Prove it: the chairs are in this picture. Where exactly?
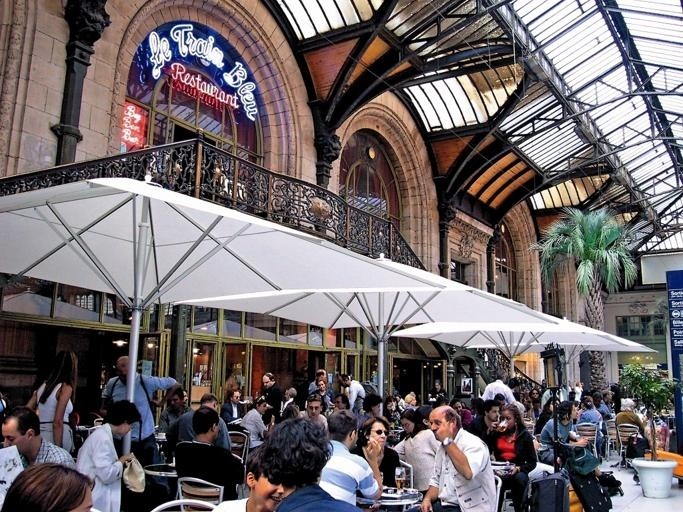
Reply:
[0,376,640,512]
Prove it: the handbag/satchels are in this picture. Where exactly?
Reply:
[363,380,378,395]
[597,471,621,496]
[626,436,650,459]
[123,453,146,493]
[575,447,600,475]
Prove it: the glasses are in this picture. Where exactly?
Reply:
[371,430,389,436]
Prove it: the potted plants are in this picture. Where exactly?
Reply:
[618,364,681,500]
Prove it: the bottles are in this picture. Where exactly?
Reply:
[203,378,210,387]
[194,373,198,385]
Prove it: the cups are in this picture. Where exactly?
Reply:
[394,466,406,495]
[372,472,383,484]
[496,419,508,432]
[394,418,398,429]
[157,433,164,438]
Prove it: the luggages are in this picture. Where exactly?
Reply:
[570,470,612,512]
[530,471,569,512]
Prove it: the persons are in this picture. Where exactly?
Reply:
[0,348,674,511]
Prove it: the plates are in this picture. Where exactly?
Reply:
[154,437,166,439]
[492,461,508,465]
